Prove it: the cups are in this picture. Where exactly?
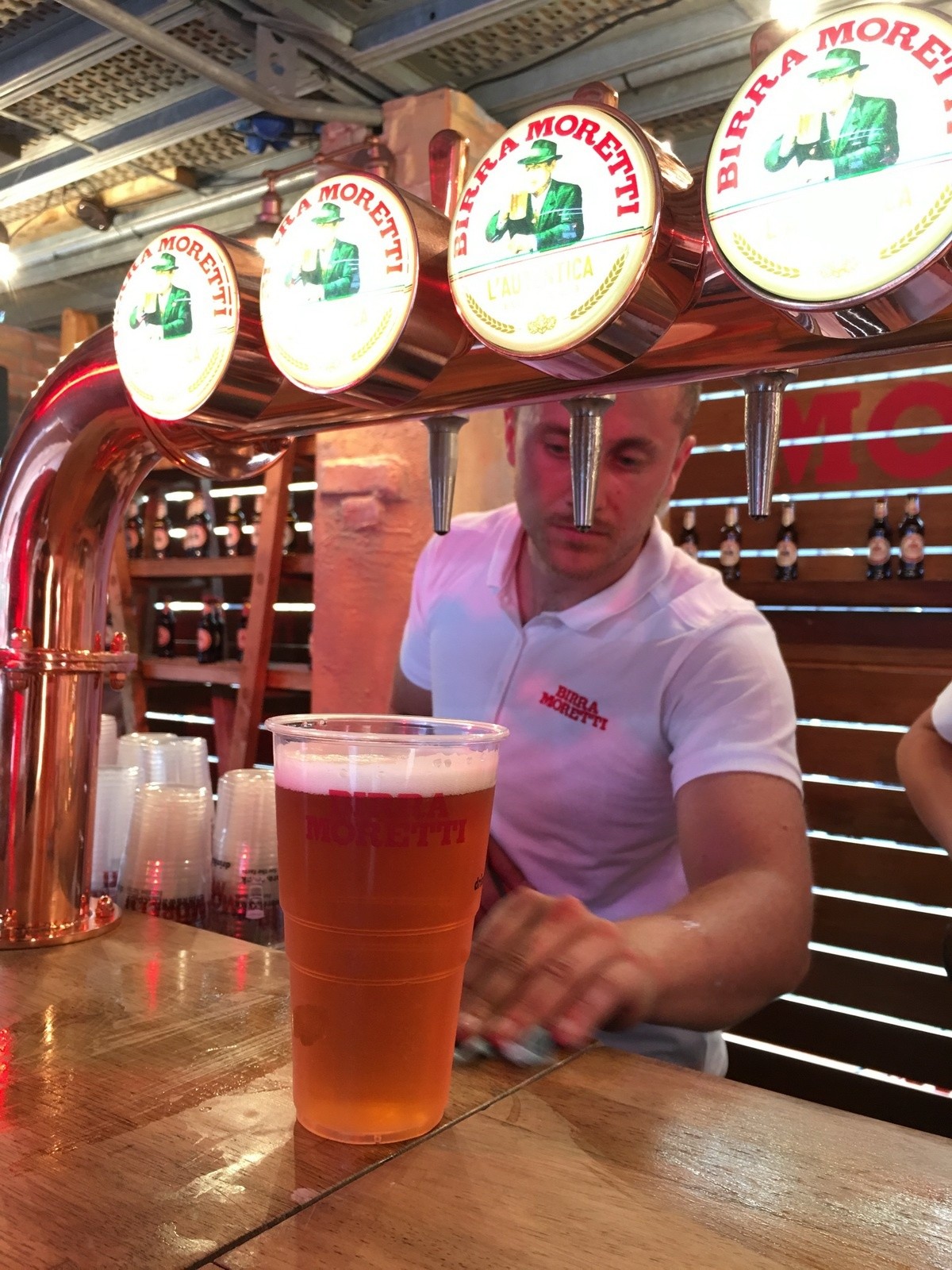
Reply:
[259,713,510,1145]
[90,714,284,951]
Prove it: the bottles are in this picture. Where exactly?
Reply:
[677,506,698,562]
[774,502,798,581]
[157,596,177,657]
[898,493,925,579]
[866,494,893,580]
[197,596,248,664]
[124,492,300,559]
[719,503,742,579]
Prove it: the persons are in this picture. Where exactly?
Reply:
[896,679,951,860]
[390,382,817,1079]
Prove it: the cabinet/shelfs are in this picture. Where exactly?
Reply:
[117,434,316,693]
